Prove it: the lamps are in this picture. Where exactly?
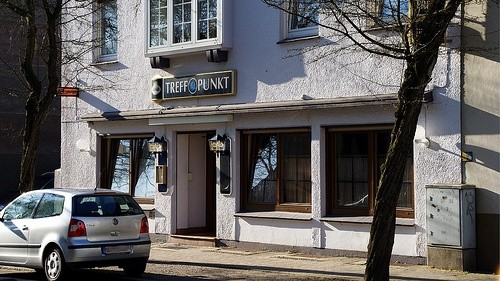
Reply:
[413,125,430,149]
[146,136,167,158]
[209,134,227,158]
[77,139,92,156]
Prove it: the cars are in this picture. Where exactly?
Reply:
[0,187,152,281]
